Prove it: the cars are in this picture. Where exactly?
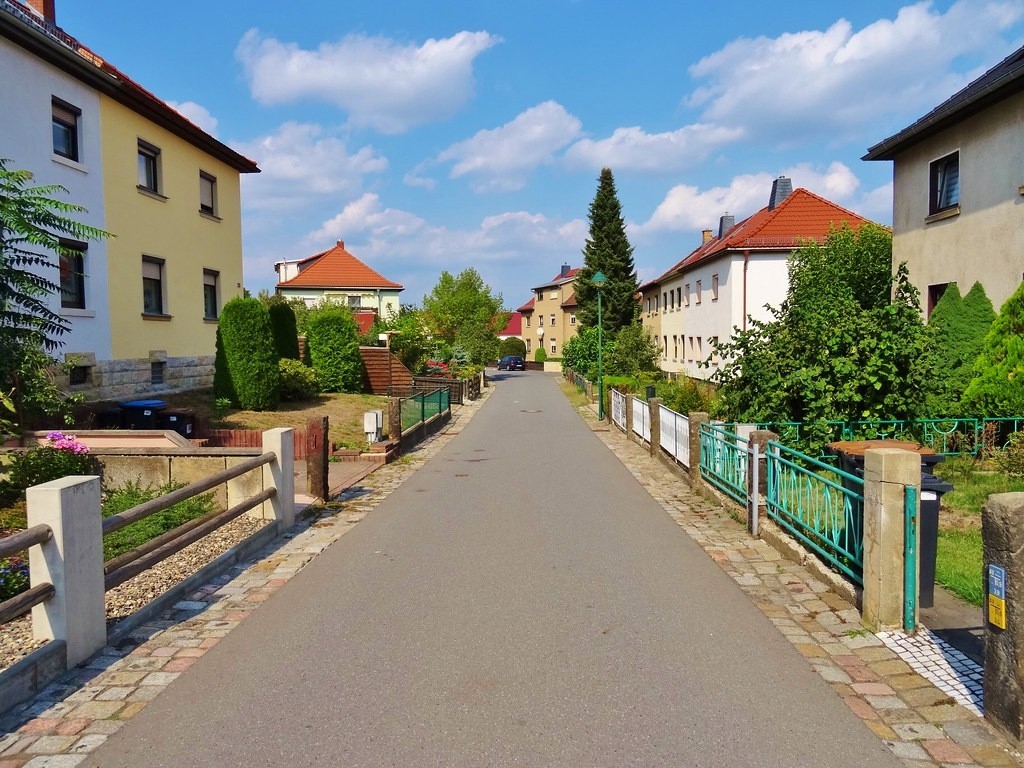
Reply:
[497,356,525,371]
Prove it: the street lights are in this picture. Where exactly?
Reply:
[592,272,607,420]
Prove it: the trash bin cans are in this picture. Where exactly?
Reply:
[855,467,955,609]
[95,408,125,430]
[158,407,195,439]
[824,438,946,592]
[119,399,167,430]
